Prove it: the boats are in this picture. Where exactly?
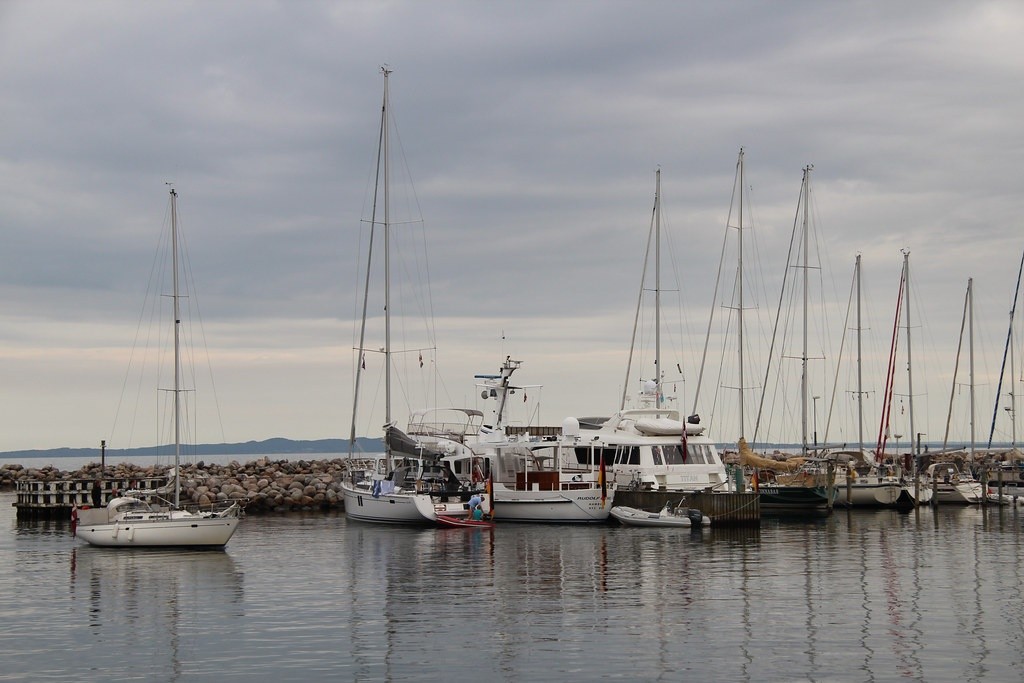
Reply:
[610,496,711,527]
[339,63,1024,525]
[72,182,241,547]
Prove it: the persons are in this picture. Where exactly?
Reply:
[487,472,495,519]
[473,506,483,520]
[92,479,101,508]
[442,467,460,502]
[467,497,485,518]
[125,480,136,491]
[108,489,117,503]
[473,461,484,481]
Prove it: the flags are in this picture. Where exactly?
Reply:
[683,422,687,463]
[598,455,607,507]
[753,470,760,493]
